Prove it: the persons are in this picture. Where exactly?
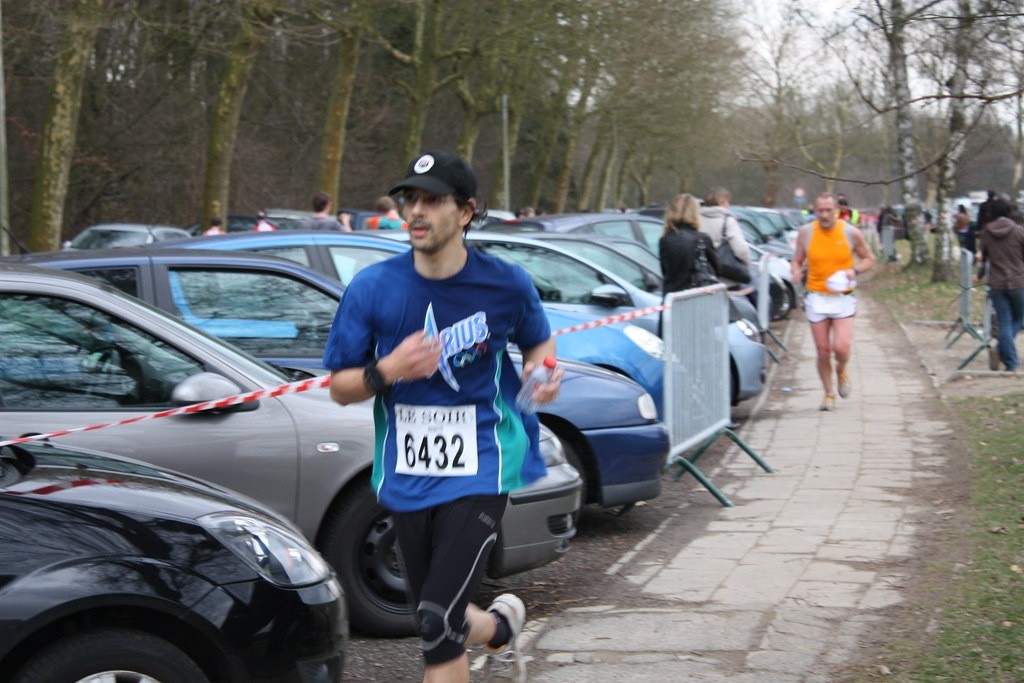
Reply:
[203,219,225,236]
[656,193,741,428]
[301,193,352,233]
[367,196,408,229]
[515,203,547,219]
[323,151,563,683]
[253,212,276,233]
[955,188,1024,372]
[791,193,876,411]
[697,186,751,295]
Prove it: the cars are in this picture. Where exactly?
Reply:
[132,229,691,432]
[482,206,818,408]
[0,252,667,531]
[63,224,190,259]
[339,207,515,230]
[204,211,344,232]
[0,260,588,631]
[0,438,349,683]
[857,192,988,226]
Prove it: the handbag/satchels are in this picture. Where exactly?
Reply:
[690,239,718,290]
[713,215,752,284]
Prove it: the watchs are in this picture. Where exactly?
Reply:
[363,360,397,395]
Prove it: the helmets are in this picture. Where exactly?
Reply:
[389,150,478,199]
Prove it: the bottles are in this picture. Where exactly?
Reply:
[516,356,556,412]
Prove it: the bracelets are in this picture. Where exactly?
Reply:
[852,267,860,276]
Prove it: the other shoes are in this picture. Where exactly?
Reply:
[989,347,1001,370]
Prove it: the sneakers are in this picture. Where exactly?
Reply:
[484,594,527,683]
[838,370,851,398]
[820,394,836,412]
